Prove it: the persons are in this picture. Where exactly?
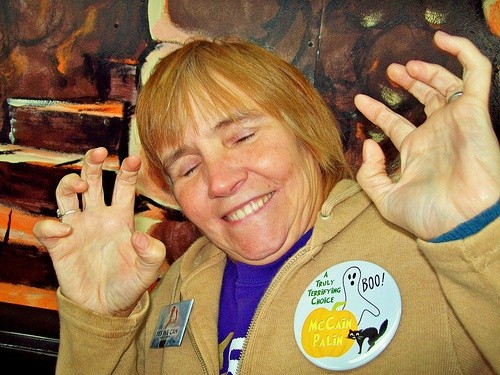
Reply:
[447,92,463,104]
[33,30,499,375]
[54,206,79,218]
